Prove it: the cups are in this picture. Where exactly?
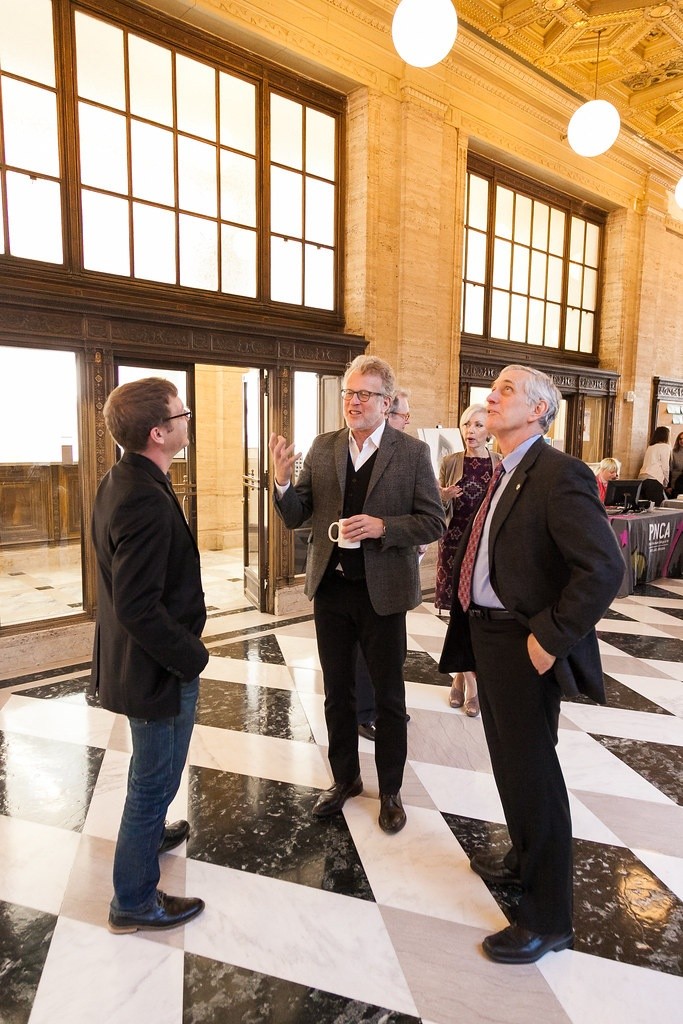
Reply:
[328,519,360,548]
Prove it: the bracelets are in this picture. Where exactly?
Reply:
[381,527,386,538]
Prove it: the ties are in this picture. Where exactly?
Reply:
[457,461,506,613]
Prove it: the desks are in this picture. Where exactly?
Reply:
[608,499,683,600]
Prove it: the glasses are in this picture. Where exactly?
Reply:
[390,410,411,421]
[340,389,386,402]
[161,406,192,423]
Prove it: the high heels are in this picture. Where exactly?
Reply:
[464,691,480,717]
[449,676,465,708]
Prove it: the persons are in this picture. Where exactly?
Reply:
[596,458,620,509]
[436,365,625,965]
[91,379,209,934]
[666,432,683,496]
[356,391,411,744]
[437,403,503,717]
[269,357,447,836]
[638,427,673,508]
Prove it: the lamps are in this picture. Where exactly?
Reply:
[392,0,458,67]
[674,174,683,209]
[567,27,620,157]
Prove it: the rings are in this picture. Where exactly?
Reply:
[361,528,364,533]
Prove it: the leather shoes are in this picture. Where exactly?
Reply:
[312,773,364,817]
[482,919,574,964]
[108,889,205,935]
[155,819,190,854]
[358,724,376,741]
[406,713,410,722]
[469,852,526,885]
[378,789,407,834]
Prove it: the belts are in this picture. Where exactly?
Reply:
[465,608,516,621]
[334,569,366,580]
